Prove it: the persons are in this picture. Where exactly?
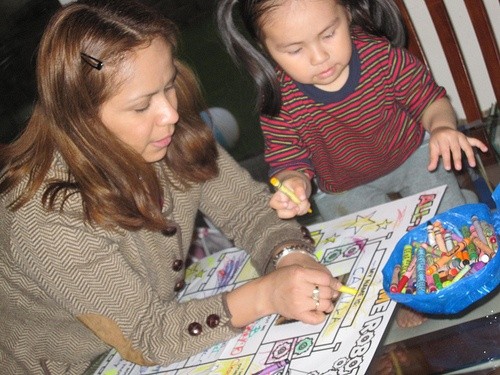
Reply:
[218,0,487,219]
[0,0,341,375]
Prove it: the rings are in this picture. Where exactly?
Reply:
[312,285,320,310]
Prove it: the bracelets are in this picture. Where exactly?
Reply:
[272,244,319,271]
